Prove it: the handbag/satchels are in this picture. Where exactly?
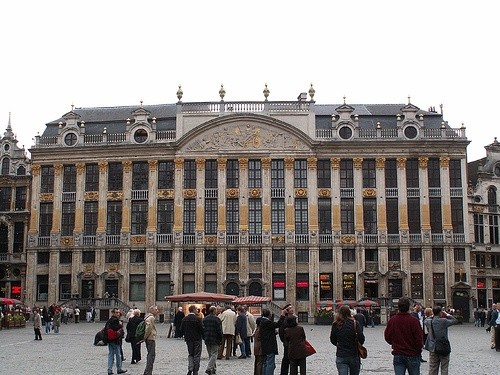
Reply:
[359,346,367,359]
[94,331,107,346]
[435,339,451,357]
[305,339,316,357]
[236,333,242,344]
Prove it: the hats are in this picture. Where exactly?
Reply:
[282,304,292,311]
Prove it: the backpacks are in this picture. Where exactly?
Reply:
[135,315,151,340]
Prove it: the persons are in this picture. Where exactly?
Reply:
[253,303,306,375]
[215,303,256,360]
[105,304,144,375]
[0,304,80,334]
[411,304,434,363]
[384,298,423,375]
[474,308,480,327]
[33,310,42,340]
[486,303,500,350]
[349,305,375,332]
[181,305,203,375]
[141,306,159,375]
[424,306,456,375]
[330,306,365,375]
[202,306,222,375]
[174,307,185,339]
[480,309,486,327]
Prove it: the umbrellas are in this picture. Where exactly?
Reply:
[317,299,379,307]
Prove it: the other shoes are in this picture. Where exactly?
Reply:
[205,370,212,375]
[217,354,251,360]
[121,357,126,361]
[117,369,127,374]
[212,371,216,374]
[474,324,484,327]
[420,356,427,362]
[131,360,136,364]
[187,370,192,375]
[108,372,114,375]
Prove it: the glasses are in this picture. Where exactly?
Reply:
[115,312,120,314]
